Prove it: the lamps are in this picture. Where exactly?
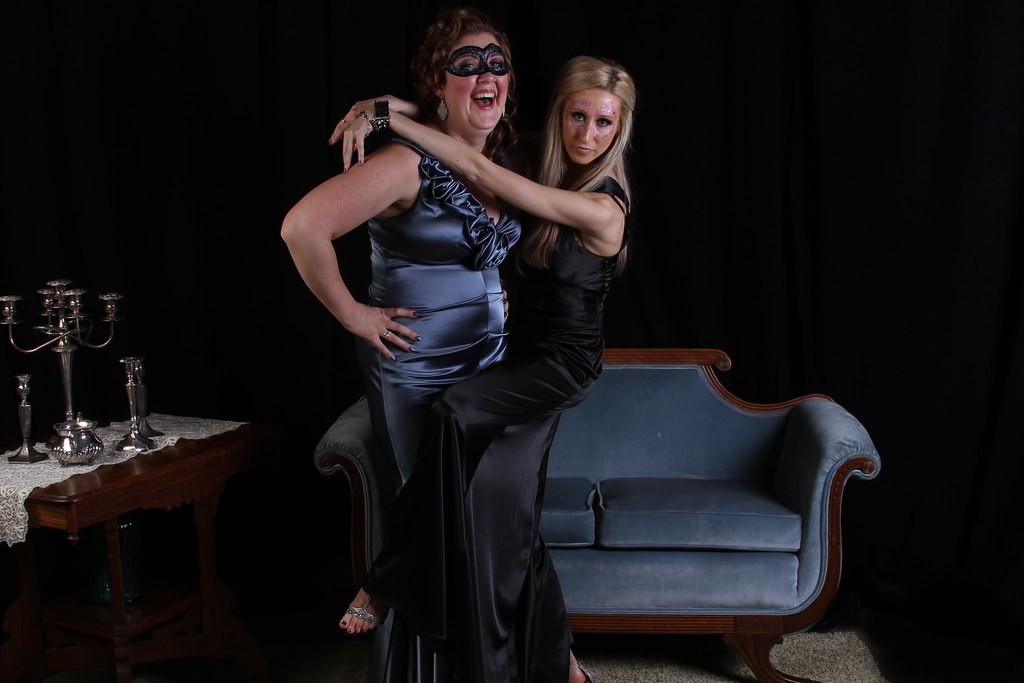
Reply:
[1,282,120,423]
[117,360,154,454]
[10,374,46,464]
[131,358,163,438]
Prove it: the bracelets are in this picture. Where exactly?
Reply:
[359,99,391,137]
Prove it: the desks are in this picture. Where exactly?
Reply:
[0,413,276,683]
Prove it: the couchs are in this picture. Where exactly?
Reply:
[314,351,880,682]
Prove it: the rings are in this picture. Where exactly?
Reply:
[342,118,346,124]
[383,330,391,341]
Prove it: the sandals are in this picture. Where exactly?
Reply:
[339,593,387,637]
[568,660,596,683]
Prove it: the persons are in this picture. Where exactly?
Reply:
[280,9,526,683]
[335,55,638,683]
